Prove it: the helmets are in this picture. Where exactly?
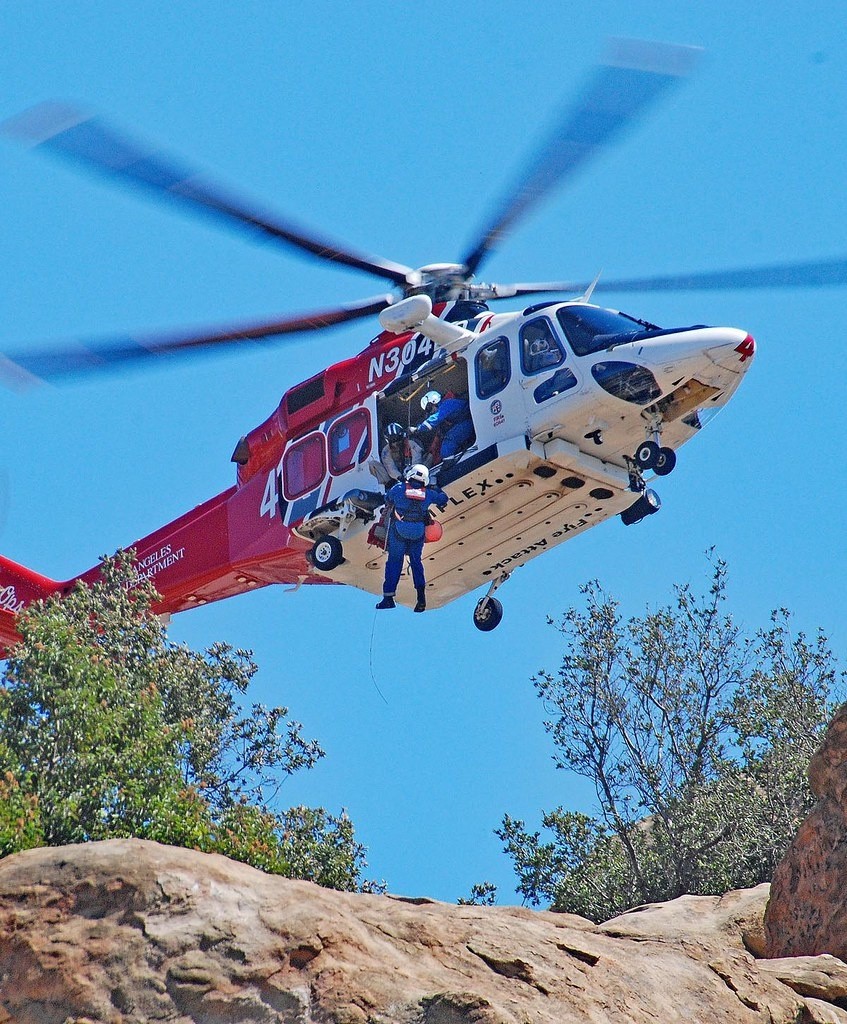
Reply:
[420,390,441,415]
[406,464,430,487]
[384,423,404,442]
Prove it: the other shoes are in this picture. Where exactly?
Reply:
[375,595,396,609]
[413,601,426,613]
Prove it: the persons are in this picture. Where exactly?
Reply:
[369,423,434,491]
[562,312,618,352]
[406,391,474,472]
[529,330,576,399]
[375,464,448,613]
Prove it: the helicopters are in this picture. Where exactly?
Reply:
[2,26,846,678]
